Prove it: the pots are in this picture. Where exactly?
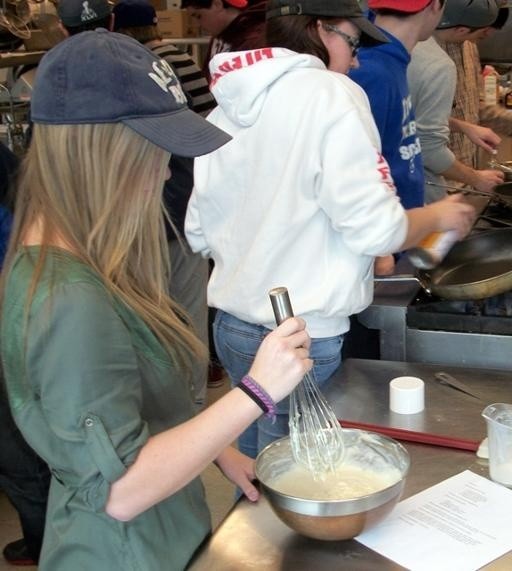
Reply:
[369,229,512,300]
[426,178,512,207]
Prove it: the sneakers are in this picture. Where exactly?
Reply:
[2,539,35,565]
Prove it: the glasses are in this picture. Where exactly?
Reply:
[320,24,360,57]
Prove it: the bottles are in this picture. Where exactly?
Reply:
[487,149,500,170]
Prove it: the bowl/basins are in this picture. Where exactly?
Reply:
[252,427,411,546]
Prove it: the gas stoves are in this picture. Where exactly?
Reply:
[401,182,508,334]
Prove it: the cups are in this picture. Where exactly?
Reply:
[482,401,512,484]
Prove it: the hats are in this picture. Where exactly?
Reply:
[368,0,431,14]
[31,29,232,157]
[58,1,110,27]
[112,0,158,27]
[264,1,393,51]
[437,2,498,31]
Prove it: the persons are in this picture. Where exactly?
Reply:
[2,4,512,407]
[2,23,318,571]
[2,141,54,568]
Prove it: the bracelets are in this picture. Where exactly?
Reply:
[241,378,274,411]
[243,375,277,408]
[236,382,269,415]
